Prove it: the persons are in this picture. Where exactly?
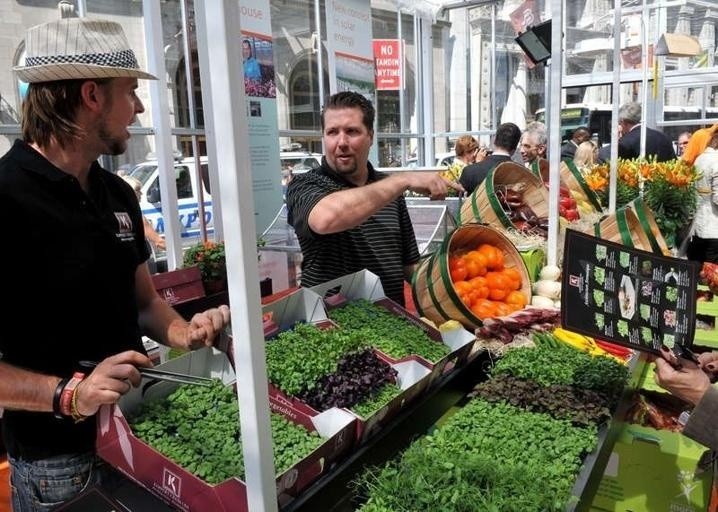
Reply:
[0,21,230,511]
[520,121,546,167]
[243,40,261,83]
[653,345,718,457]
[561,103,692,164]
[690,123,718,276]
[456,124,520,197]
[286,91,465,311]
[124,177,167,251]
[446,133,488,186]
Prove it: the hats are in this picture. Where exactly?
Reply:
[12,17,160,83]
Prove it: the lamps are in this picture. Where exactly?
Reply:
[657,32,702,56]
[514,19,565,66]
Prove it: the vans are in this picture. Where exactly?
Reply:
[121,151,320,255]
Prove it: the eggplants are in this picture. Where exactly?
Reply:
[474,304,562,344]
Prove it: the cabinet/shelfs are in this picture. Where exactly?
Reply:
[50,219,716,512]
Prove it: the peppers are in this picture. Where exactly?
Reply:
[557,185,582,224]
[446,242,531,320]
[497,183,550,241]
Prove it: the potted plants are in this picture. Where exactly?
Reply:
[183,236,265,293]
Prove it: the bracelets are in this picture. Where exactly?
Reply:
[52,362,96,424]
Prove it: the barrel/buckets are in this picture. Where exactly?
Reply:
[410,222,532,335]
[456,160,550,239]
[525,159,550,186]
[583,196,670,255]
[559,160,603,216]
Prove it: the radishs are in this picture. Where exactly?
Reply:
[530,262,561,311]
